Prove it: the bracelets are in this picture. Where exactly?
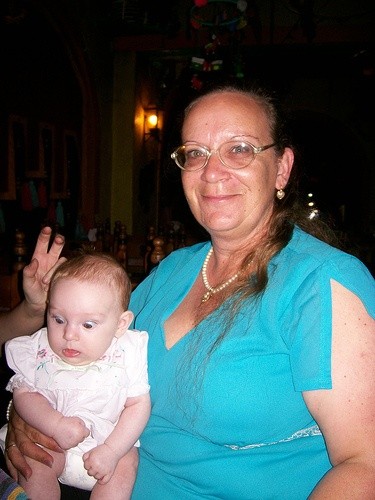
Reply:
[3,396,17,428]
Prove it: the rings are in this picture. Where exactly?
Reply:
[3,442,17,452]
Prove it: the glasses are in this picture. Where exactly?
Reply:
[170,142,278,173]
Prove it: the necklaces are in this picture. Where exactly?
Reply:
[200,246,263,303]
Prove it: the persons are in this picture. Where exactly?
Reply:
[3,223,71,346]
[1,79,374,499]
[5,250,154,498]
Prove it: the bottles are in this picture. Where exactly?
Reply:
[9,232,30,310]
[73,220,191,279]
